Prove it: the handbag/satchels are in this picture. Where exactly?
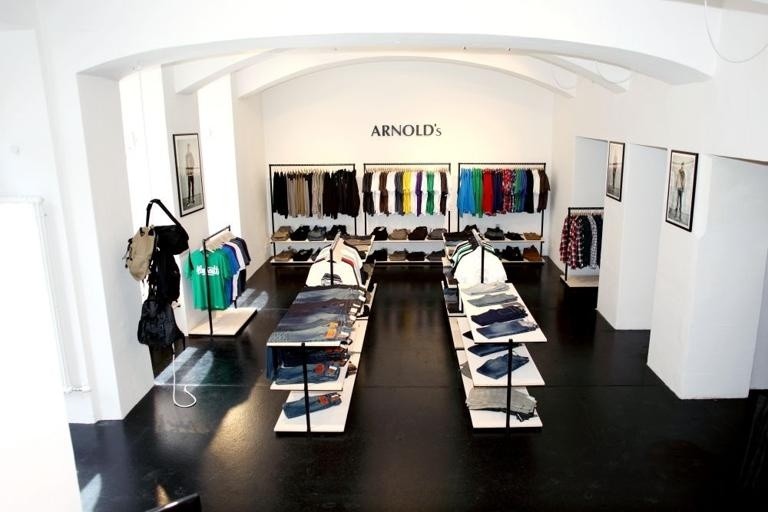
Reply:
[144,198,191,255]
[134,298,185,352]
[124,225,157,283]
[148,252,181,305]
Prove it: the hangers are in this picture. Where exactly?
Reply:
[199,228,237,253]
[570,207,604,218]
[460,162,546,176]
[364,162,451,174]
[273,163,355,176]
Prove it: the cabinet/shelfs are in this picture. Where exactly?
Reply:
[440,231,548,440]
[272,232,381,441]
[381,232,440,266]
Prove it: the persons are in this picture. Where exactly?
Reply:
[609,147,619,193]
[673,161,686,222]
[183,143,197,208]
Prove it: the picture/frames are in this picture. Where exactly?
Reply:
[605,140,625,202]
[173,132,205,217]
[665,149,700,232]
